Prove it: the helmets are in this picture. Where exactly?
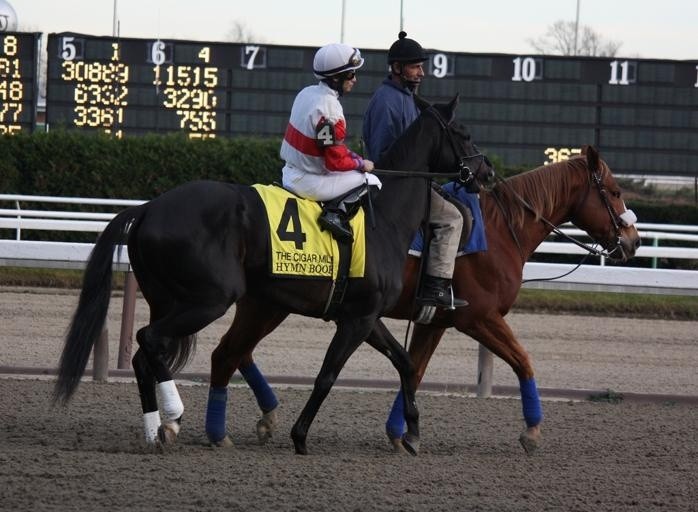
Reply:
[388,31,429,64]
[313,43,365,80]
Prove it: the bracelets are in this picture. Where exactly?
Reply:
[356,157,365,171]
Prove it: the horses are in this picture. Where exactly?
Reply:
[205,142,646,460]
[54,91,496,458]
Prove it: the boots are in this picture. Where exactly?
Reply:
[318,183,371,242]
[419,275,469,306]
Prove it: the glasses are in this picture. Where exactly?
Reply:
[320,71,355,80]
[313,48,361,73]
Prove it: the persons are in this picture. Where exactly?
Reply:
[279,41,383,244]
[361,31,470,309]
